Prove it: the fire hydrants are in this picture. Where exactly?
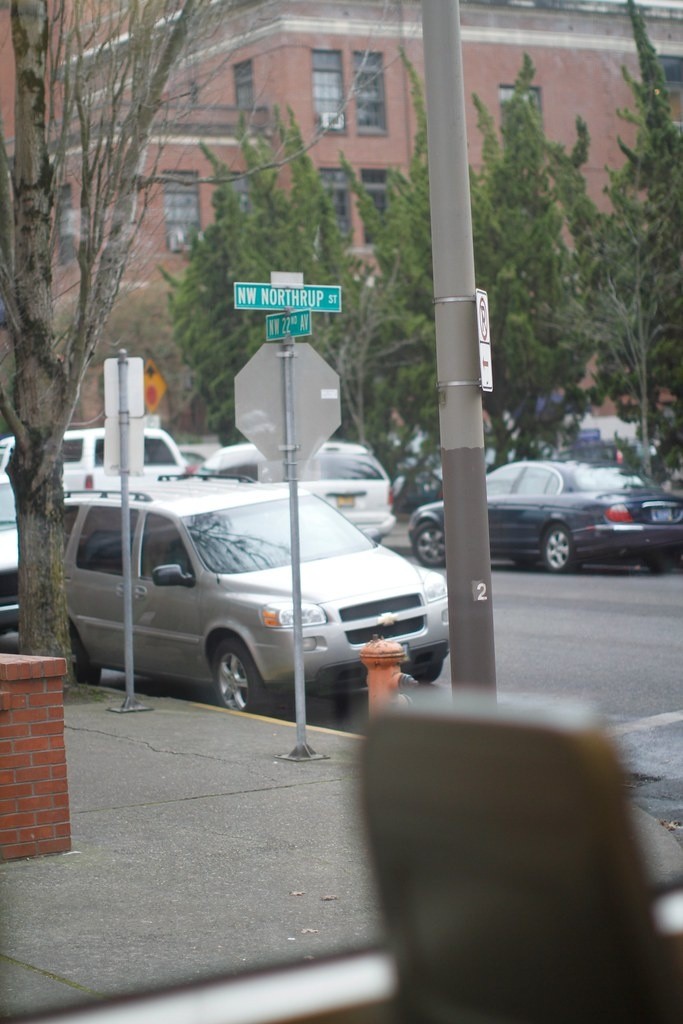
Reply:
[359,634,420,721]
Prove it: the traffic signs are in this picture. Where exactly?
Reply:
[264,308,312,342]
[233,280,342,313]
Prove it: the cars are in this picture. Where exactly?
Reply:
[408,454,683,571]
[188,437,397,545]
[0,474,20,634]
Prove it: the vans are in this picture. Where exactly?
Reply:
[0,425,194,488]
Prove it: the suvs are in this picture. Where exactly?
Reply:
[60,474,450,713]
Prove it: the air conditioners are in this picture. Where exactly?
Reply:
[172,232,204,252]
[321,112,345,129]
